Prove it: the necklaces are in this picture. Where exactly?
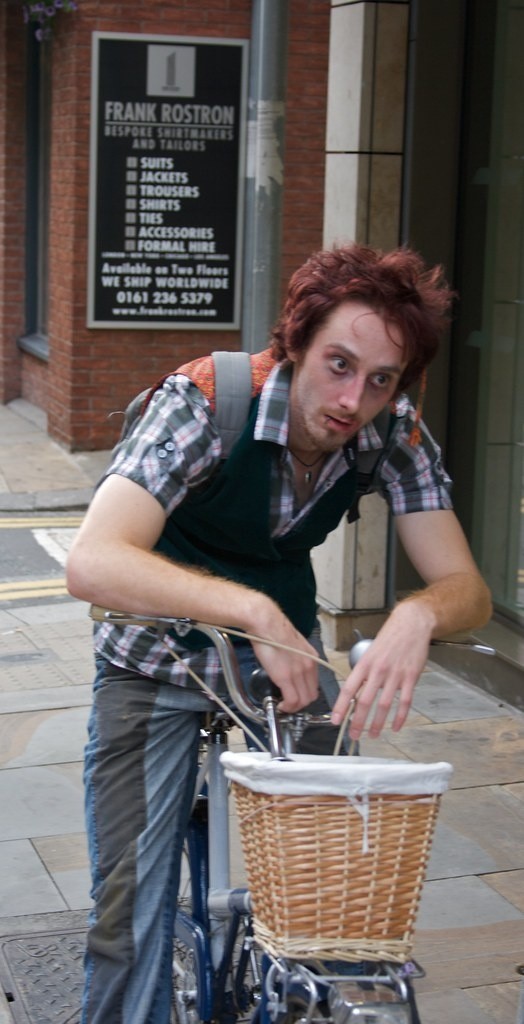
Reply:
[288,448,324,484]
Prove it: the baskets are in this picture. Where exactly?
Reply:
[219,742,452,964]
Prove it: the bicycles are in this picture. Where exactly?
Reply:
[84,599,456,1024]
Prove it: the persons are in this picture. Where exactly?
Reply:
[66,243,492,1024]
